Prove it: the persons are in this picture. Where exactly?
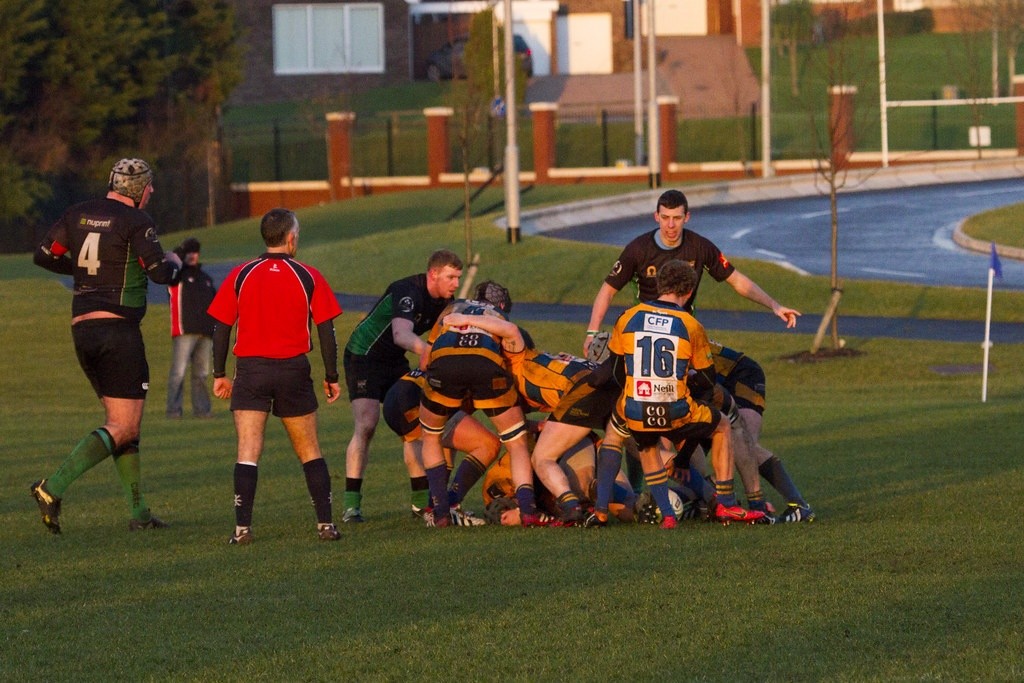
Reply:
[167,238,217,420]
[207,209,342,544]
[343,189,815,527]
[30,157,187,535]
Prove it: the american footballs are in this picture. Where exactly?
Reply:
[655,488,684,523]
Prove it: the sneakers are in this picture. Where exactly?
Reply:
[228,526,253,545]
[549,505,583,527]
[714,503,766,527]
[570,511,607,528]
[30,477,64,537]
[519,512,561,529]
[749,511,781,524]
[411,503,432,522]
[425,515,451,528]
[702,492,718,523]
[450,504,485,526]
[316,522,339,540]
[129,508,169,532]
[778,502,815,524]
[657,516,679,530]
[342,507,365,523]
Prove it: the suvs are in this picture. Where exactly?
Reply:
[425,31,534,84]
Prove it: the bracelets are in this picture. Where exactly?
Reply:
[584,329,599,338]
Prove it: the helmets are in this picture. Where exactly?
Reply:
[475,280,512,314]
[587,332,613,365]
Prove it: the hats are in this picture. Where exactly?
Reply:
[107,158,154,200]
[181,239,200,253]
